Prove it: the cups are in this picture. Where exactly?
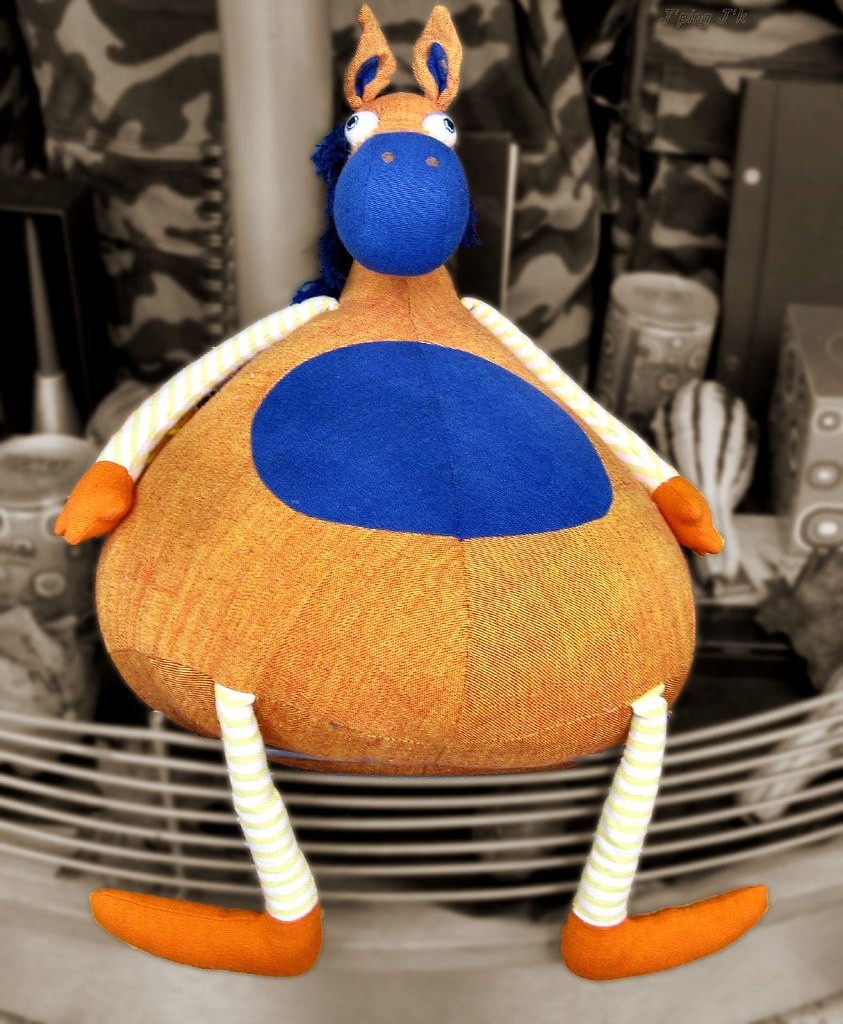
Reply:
[0,433,108,633]
[598,269,720,429]
